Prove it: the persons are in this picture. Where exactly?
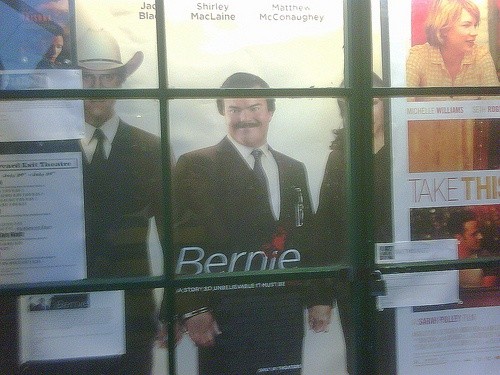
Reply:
[169,72,334,375]
[75,29,185,375]
[439,210,497,290]
[316,69,390,375]
[406,0,499,88]
[25,21,70,66]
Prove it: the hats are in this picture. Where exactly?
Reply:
[78,26,143,78]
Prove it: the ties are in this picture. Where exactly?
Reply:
[251,149,269,208]
[89,129,108,170]
